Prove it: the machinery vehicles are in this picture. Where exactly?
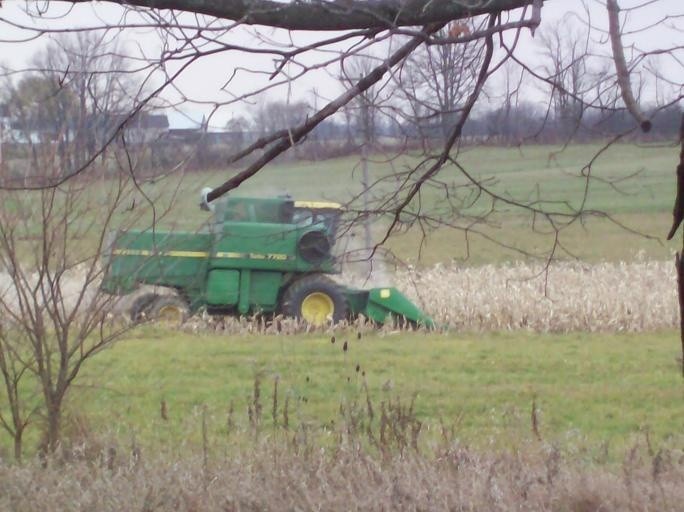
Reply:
[97,191,435,337]
